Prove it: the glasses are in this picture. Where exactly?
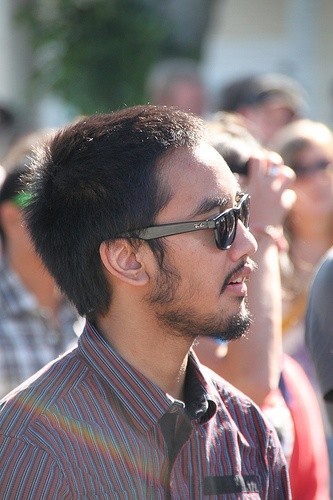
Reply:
[114,190,250,250]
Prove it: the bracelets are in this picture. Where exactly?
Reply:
[250,222,289,252]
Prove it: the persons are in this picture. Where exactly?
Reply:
[0,106,292,500]
[0,130,87,401]
[266,119,333,500]
[190,128,329,500]
[223,71,307,146]
